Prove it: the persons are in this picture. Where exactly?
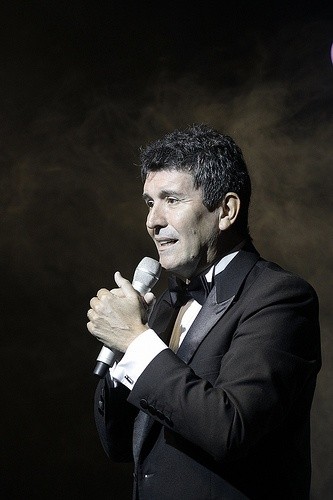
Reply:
[88,126,322,500]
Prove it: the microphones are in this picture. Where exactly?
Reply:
[92,257,162,378]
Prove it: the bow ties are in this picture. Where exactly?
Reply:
[167,271,210,307]
